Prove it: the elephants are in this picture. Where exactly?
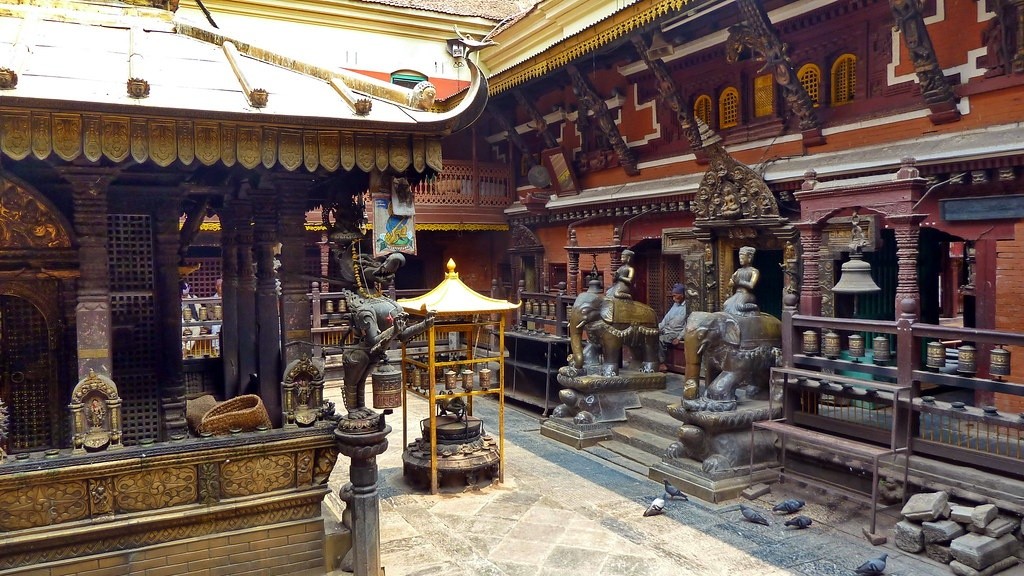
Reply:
[566,292,659,376]
[683,312,783,399]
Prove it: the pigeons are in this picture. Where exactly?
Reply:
[784,516,812,528]
[855,554,889,573]
[739,504,769,526]
[662,478,688,501]
[643,492,666,516]
[772,499,805,513]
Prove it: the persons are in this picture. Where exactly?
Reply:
[413,81,436,109]
[90,399,102,427]
[298,381,309,405]
[182,282,207,359]
[605,249,635,300]
[249,88,269,106]
[354,98,372,113]
[126,77,150,97]
[720,187,737,211]
[658,283,687,373]
[502,0,929,155]
[723,246,761,317]
[211,278,222,356]
[0,66,18,88]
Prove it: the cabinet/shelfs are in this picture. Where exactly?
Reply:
[751,368,913,535]
[494,332,571,418]
